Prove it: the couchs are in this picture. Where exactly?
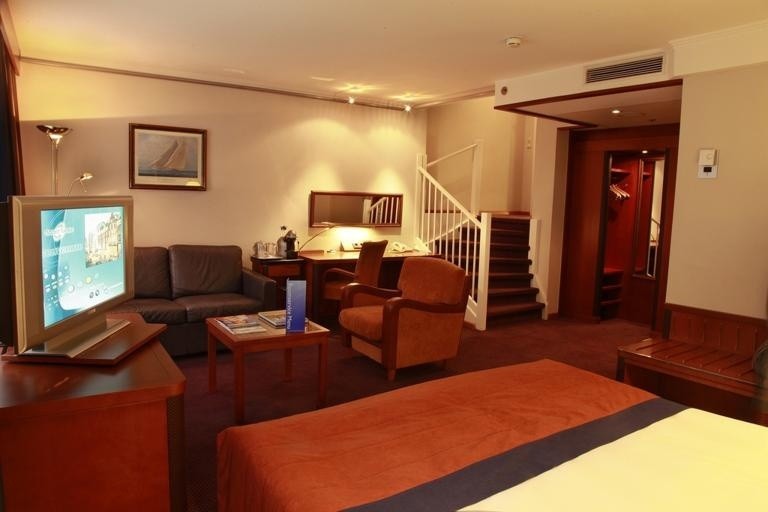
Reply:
[129,243,278,358]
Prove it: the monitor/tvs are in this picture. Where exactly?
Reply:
[0,195,135,359]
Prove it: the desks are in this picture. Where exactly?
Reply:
[251,255,304,279]
[297,249,442,322]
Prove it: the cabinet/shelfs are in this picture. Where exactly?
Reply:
[600,268,624,305]
[0,312,190,511]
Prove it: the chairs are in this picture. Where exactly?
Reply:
[321,239,472,388]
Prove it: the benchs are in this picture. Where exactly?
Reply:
[616,303,767,403]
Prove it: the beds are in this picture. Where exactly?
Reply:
[214,357,767,512]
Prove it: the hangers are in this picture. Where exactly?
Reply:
[609,183,631,201]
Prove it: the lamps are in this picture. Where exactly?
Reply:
[37,124,94,195]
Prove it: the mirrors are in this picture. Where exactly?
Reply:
[310,191,403,228]
[630,155,666,280]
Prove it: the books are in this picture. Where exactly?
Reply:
[258,308,309,329]
[214,314,266,335]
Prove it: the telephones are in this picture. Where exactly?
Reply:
[392,242,413,253]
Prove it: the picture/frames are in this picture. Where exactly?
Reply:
[128,122,208,192]
